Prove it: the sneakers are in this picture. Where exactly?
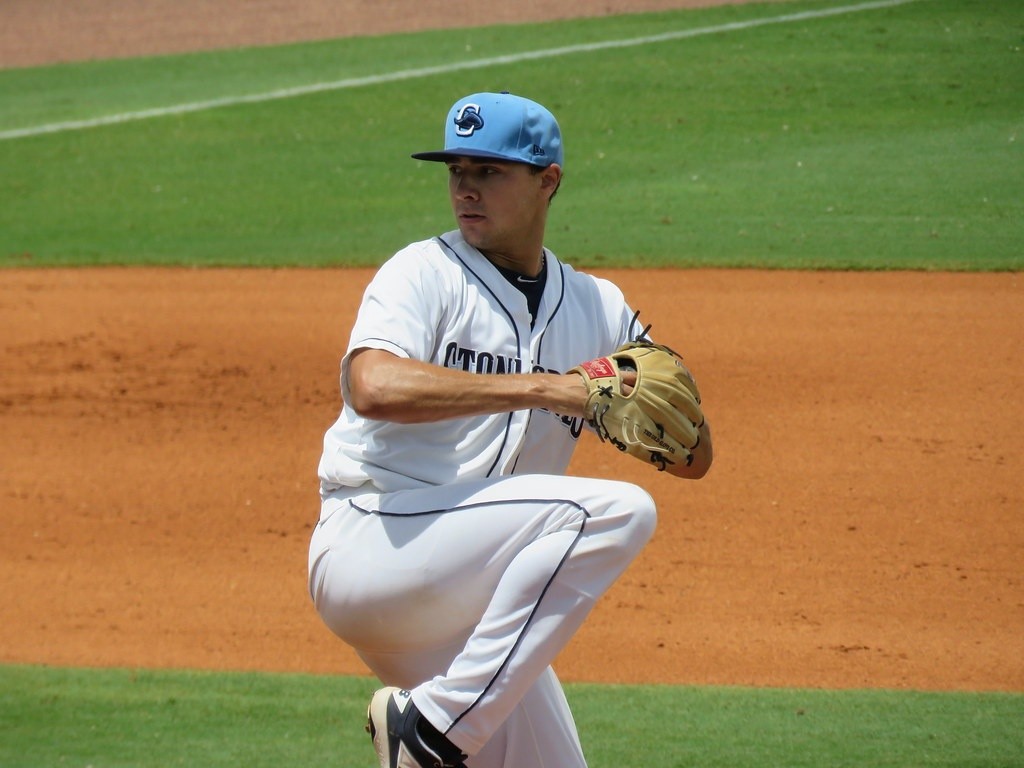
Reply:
[365,688,468,768]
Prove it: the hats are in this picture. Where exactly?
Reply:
[410,92,563,170]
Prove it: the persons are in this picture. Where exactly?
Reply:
[305,92,713,768]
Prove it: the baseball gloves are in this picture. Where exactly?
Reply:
[564,342,703,471]
